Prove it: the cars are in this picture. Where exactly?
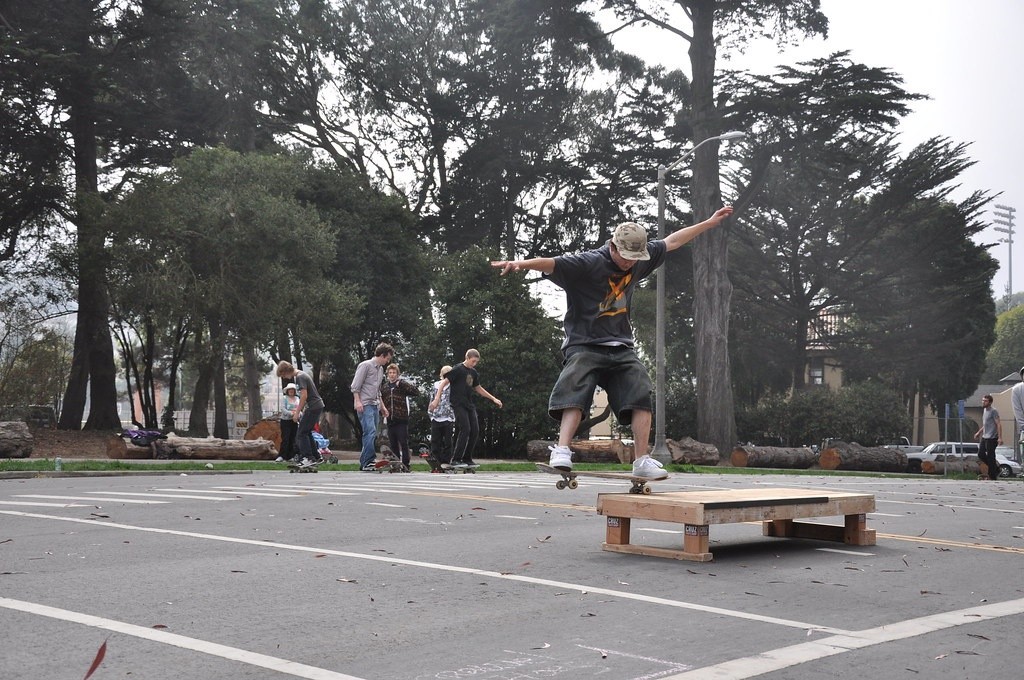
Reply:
[806,433,1023,478]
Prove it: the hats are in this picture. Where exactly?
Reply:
[612,222,651,261]
[283,383,297,394]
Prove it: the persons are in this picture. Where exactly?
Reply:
[276,383,300,462]
[277,360,325,468]
[974,395,1004,480]
[491,206,733,480]
[381,363,420,473]
[351,343,394,471]
[428,366,455,463]
[431,349,502,466]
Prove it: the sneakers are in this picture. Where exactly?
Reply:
[451,460,468,467]
[548,443,575,471]
[633,457,668,479]
[464,460,479,468]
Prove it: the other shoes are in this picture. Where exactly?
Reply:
[361,459,379,471]
[288,457,294,461]
[315,457,325,464]
[276,456,283,462]
[296,457,317,467]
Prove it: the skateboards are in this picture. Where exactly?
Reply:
[380,444,408,473]
[417,443,446,473]
[440,463,481,474]
[363,460,390,473]
[287,465,318,473]
[533,462,671,495]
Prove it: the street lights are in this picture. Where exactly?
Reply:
[650,130,750,464]
[994,202,1018,312]
